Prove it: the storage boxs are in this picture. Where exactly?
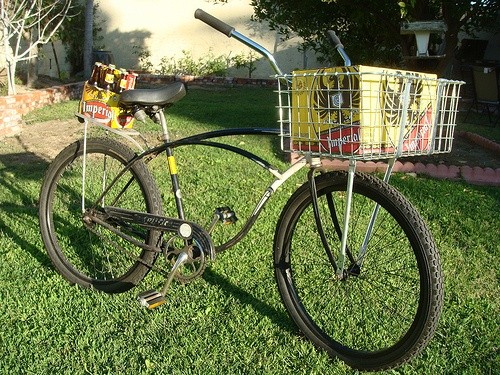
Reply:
[289,65,438,156]
[78,80,136,130]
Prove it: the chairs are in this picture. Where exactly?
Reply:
[463,66,500,127]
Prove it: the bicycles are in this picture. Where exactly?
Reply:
[38,8,468,371]
